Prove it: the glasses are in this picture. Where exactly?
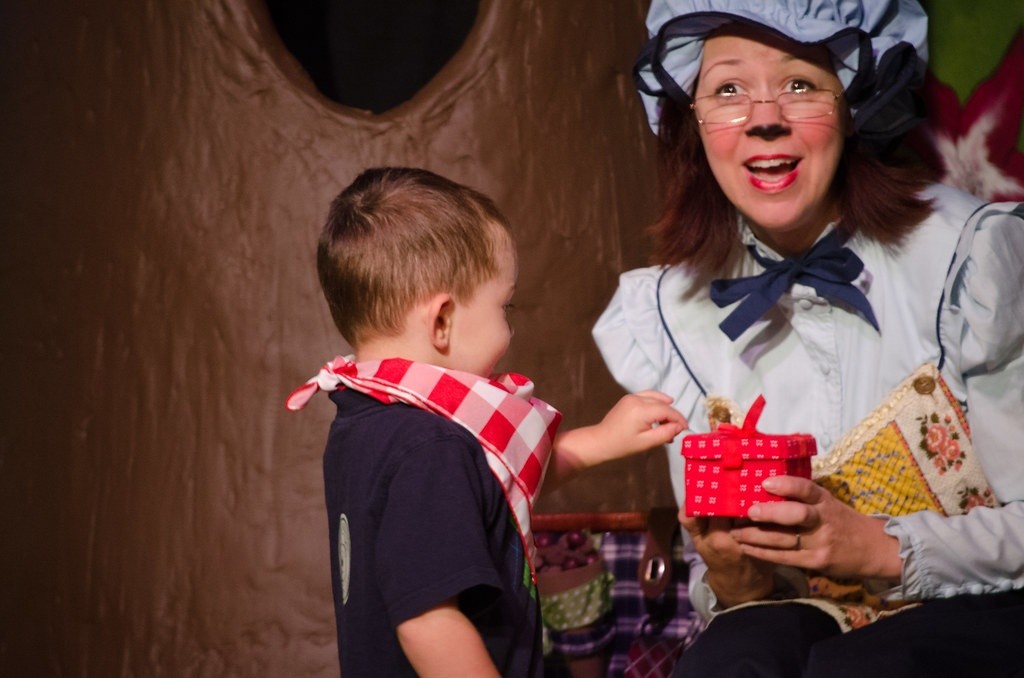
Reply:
[688,88,850,126]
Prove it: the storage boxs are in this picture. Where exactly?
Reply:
[680,431,817,519]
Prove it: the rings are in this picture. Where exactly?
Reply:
[795,533,801,549]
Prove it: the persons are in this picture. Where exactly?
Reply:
[591,0,1024,678]
[315,166,689,678]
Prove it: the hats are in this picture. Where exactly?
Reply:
[632,1,931,137]
